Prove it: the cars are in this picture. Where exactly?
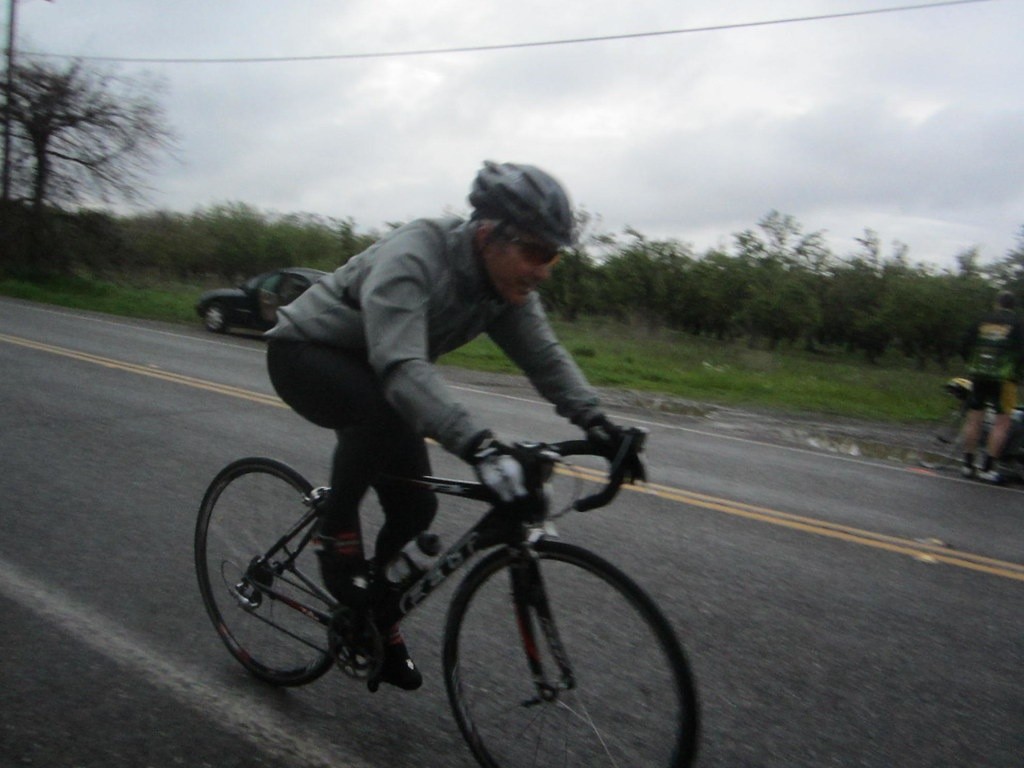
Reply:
[194,268,336,334]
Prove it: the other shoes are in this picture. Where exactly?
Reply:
[960,464,999,481]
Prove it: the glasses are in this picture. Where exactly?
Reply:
[511,234,559,266]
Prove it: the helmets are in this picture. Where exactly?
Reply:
[471,163,574,253]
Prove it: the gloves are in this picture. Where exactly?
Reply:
[593,419,644,476]
[472,442,550,524]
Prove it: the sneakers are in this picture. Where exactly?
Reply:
[314,509,366,601]
[374,625,425,693]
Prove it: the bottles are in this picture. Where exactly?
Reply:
[385,532,443,584]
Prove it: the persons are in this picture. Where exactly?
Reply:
[262,160,649,693]
[960,292,1024,483]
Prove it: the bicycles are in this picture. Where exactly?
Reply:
[923,374,1023,486]
[192,423,701,768]
